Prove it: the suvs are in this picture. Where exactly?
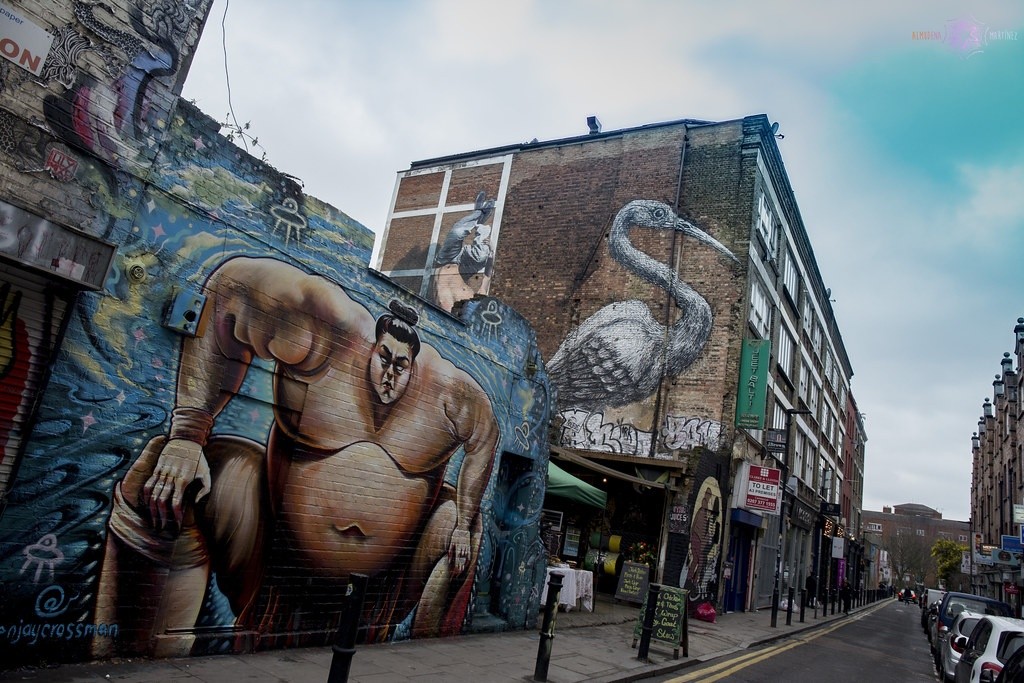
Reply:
[930,591,1013,666]
[921,589,958,629]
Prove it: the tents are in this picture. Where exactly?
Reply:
[546,460,607,612]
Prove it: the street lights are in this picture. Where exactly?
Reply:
[770,410,813,627]
[961,521,973,593]
[857,529,872,606]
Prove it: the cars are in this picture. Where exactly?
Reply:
[925,600,966,642]
[939,610,987,683]
[898,589,916,603]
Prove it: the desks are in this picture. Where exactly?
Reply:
[539,567,593,614]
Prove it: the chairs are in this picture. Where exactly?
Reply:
[948,604,964,619]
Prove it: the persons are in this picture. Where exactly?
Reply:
[903,586,911,607]
[806,571,817,609]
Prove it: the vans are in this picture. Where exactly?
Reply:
[953,615,1024,683]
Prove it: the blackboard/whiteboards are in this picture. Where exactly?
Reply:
[635,582,688,649]
[615,560,652,603]
